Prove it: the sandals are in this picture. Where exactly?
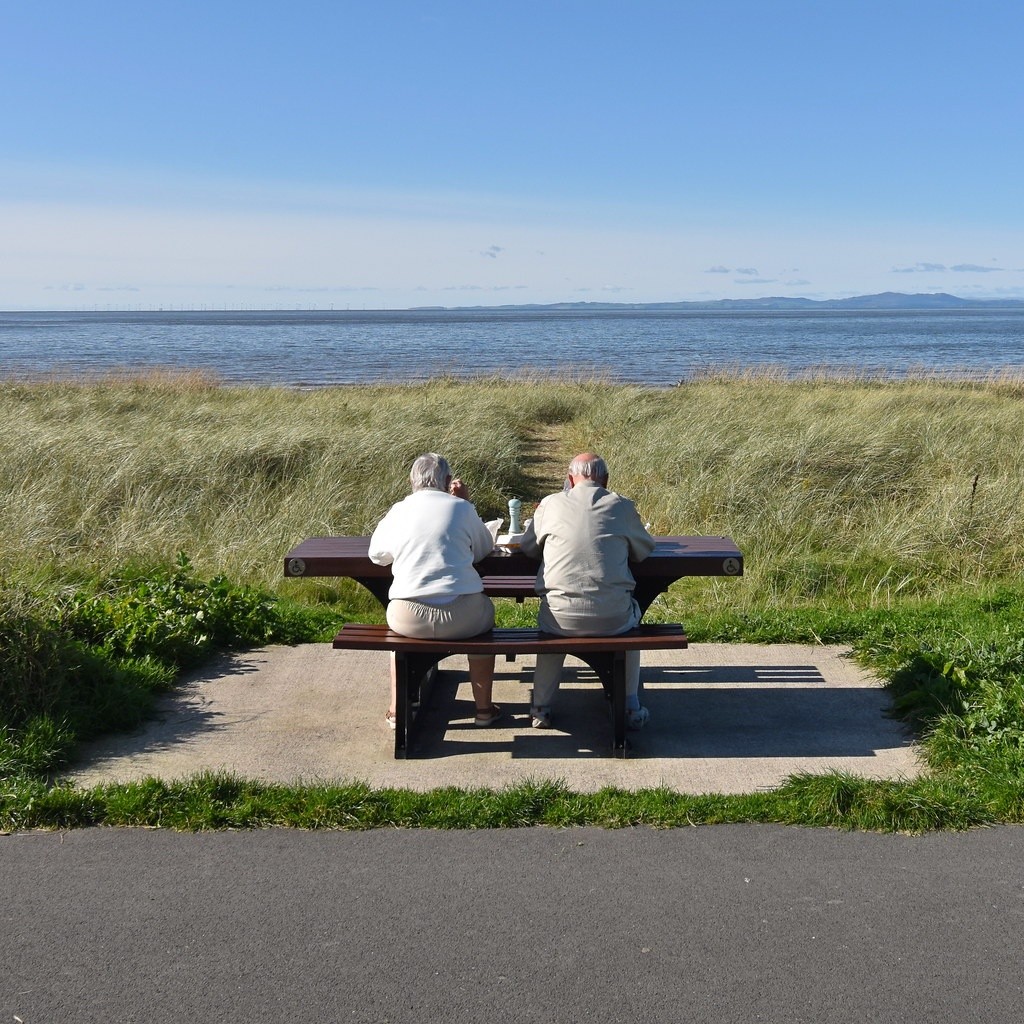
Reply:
[474,703,504,726]
[384,710,397,731]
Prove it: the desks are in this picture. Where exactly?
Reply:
[283,531,744,713]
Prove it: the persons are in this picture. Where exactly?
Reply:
[367,449,504,729]
[521,452,656,731]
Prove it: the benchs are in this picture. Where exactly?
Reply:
[478,575,542,604]
[331,620,689,761]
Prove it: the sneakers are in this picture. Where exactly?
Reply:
[627,706,651,730]
[529,705,552,728]
